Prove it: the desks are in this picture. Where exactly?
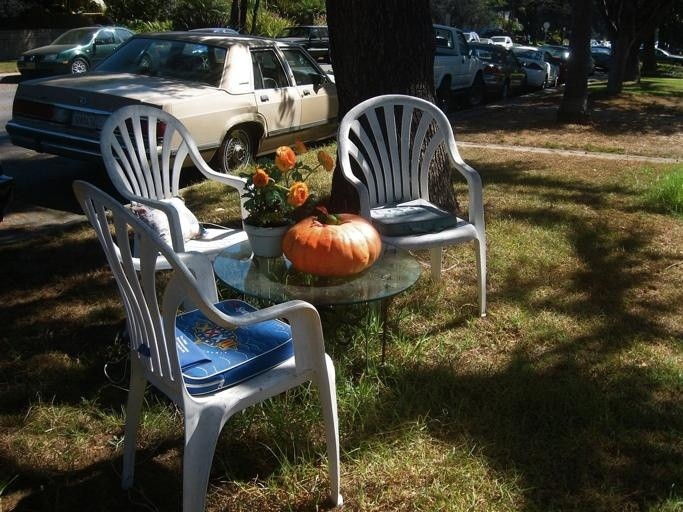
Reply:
[212,237,420,366]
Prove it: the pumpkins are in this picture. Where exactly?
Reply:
[283,213,381,275]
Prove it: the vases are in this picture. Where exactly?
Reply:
[243,218,293,257]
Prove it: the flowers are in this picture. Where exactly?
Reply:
[237,138,334,222]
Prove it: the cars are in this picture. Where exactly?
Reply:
[3,29,342,177]
[15,24,161,77]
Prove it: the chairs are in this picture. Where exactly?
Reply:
[98,104,250,260]
[70,178,345,511]
[337,94,487,320]
[436,38,448,45]
